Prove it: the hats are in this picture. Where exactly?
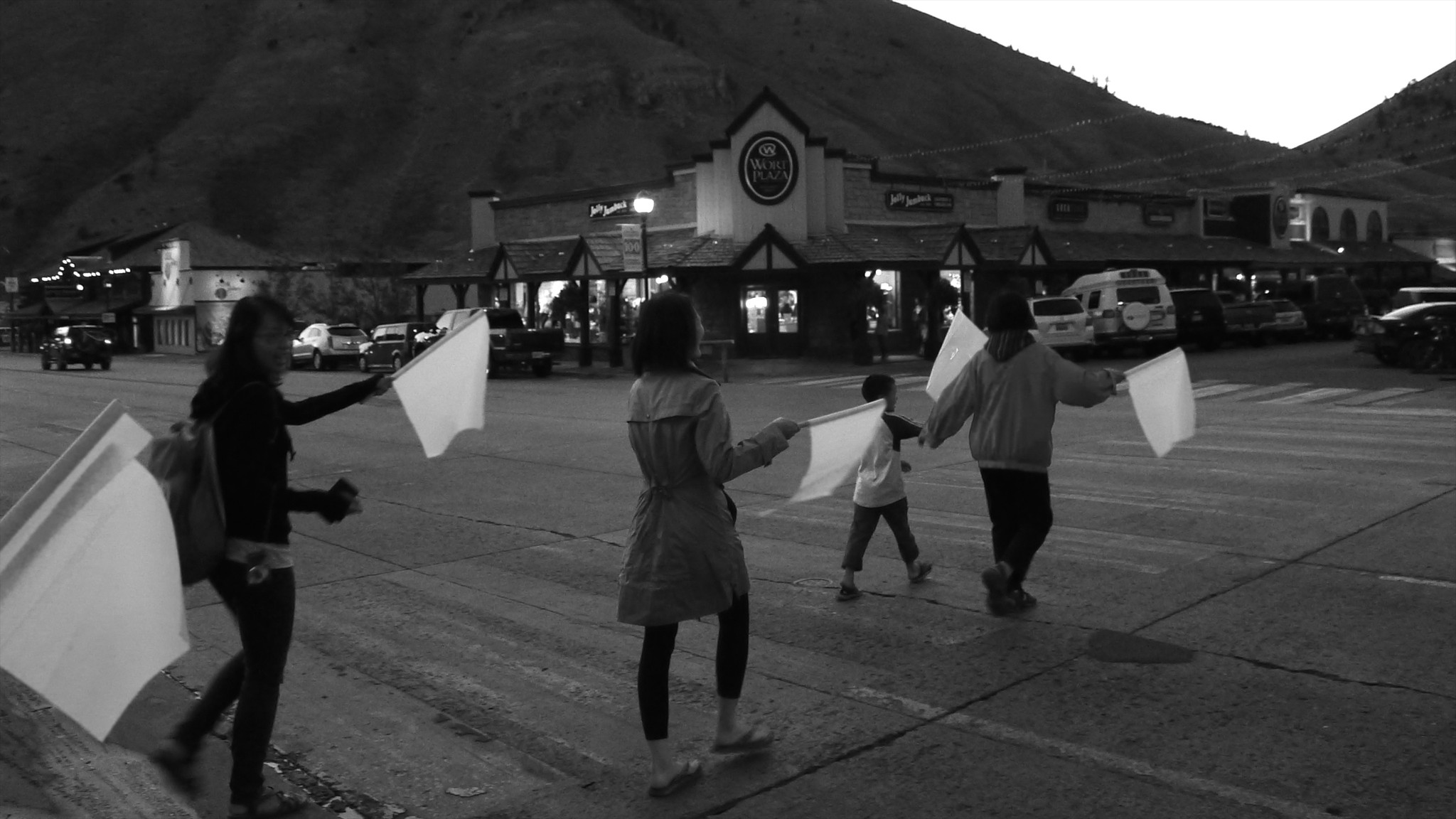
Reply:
[987,291,1038,332]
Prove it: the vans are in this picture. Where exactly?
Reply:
[1059,267,1178,362]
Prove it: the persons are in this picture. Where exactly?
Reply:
[837,373,934,601]
[875,292,929,361]
[615,291,801,799]
[916,289,1127,619]
[145,295,397,819]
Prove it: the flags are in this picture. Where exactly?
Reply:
[925,308,990,403]
[391,310,491,459]
[789,398,889,504]
[1,397,194,743]
[1123,346,1197,458]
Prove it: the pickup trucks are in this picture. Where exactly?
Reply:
[411,308,566,379]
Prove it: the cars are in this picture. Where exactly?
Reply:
[1169,268,1456,376]
[1026,295,1095,351]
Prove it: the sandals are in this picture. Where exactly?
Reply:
[1002,589,1037,611]
[152,739,188,766]
[226,785,308,819]
[981,563,1008,616]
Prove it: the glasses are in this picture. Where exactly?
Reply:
[255,329,297,347]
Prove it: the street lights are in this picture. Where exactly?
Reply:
[633,189,656,301]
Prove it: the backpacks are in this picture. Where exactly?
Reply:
[144,419,226,583]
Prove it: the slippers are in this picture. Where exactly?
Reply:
[650,759,702,795]
[838,585,863,598]
[710,726,775,753]
[911,561,933,582]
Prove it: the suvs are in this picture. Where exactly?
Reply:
[38,324,114,372]
[287,321,369,372]
[358,321,438,374]
